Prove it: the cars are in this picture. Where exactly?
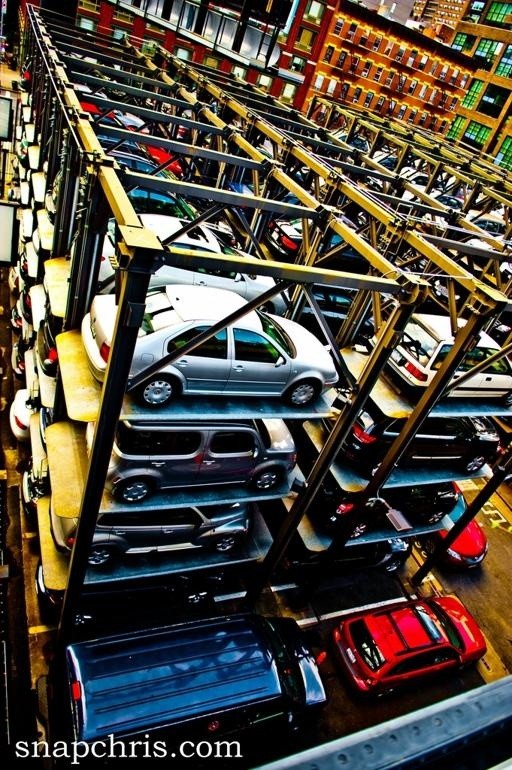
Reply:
[334,594,490,703]
[6,31,512,624]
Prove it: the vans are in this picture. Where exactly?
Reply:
[48,607,328,770]
